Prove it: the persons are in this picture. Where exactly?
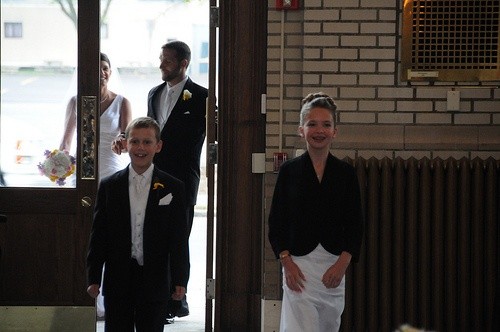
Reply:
[143,40,218,325]
[267,92,363,332]
[87,117,191,332]
[57,52,133,321]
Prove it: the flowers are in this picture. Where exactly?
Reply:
[153,183,169,197]
[36,149,76,186]
[182,89,192,112]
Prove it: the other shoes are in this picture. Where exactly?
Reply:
[178,300,189,316]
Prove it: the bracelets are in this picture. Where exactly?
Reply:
[279,252,292,261]
[118,132,127,139]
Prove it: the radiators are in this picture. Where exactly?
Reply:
[339,156,500,332]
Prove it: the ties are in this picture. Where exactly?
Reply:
[160,86,173,129]
[135,175,144,195]
